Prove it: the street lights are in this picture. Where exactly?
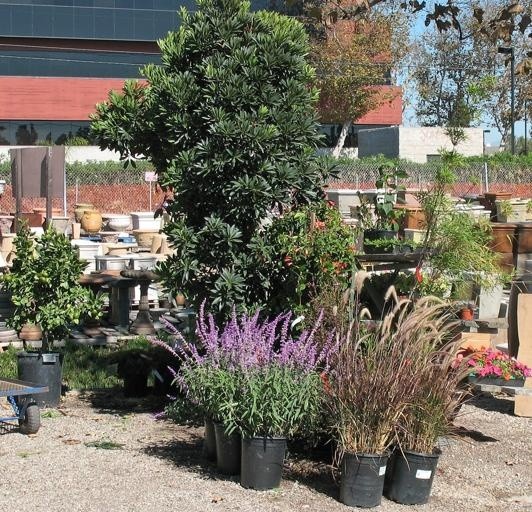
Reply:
[483,129,492,156]
[497,44,515,157]
[525,97,532,156]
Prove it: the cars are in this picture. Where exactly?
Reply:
[0,179,5,196]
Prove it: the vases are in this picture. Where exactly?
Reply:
[200,417,287,493]
[0,205,211,342]
[459,309,475,320]
[461,371,525,389]
[327,184,532,281]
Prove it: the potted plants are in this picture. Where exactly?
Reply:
[0,213,109,409]
[383,302,490,506]
[320,284,428,509]
[360,161,410,255]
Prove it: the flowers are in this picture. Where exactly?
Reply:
[142,286,348,436]
[457,344,532,382]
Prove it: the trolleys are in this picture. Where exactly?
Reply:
[0,373,53,439]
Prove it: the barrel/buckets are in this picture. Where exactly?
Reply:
[16,350,65,409]
[196,417,444,507]
